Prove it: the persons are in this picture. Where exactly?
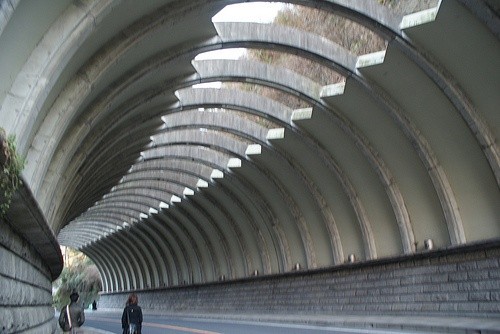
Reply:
[59,292,85,334]
[121,294,143,334]
[92,300,96,310]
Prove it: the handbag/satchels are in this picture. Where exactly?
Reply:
[71,327,83,334]
[123,324,137,334]
